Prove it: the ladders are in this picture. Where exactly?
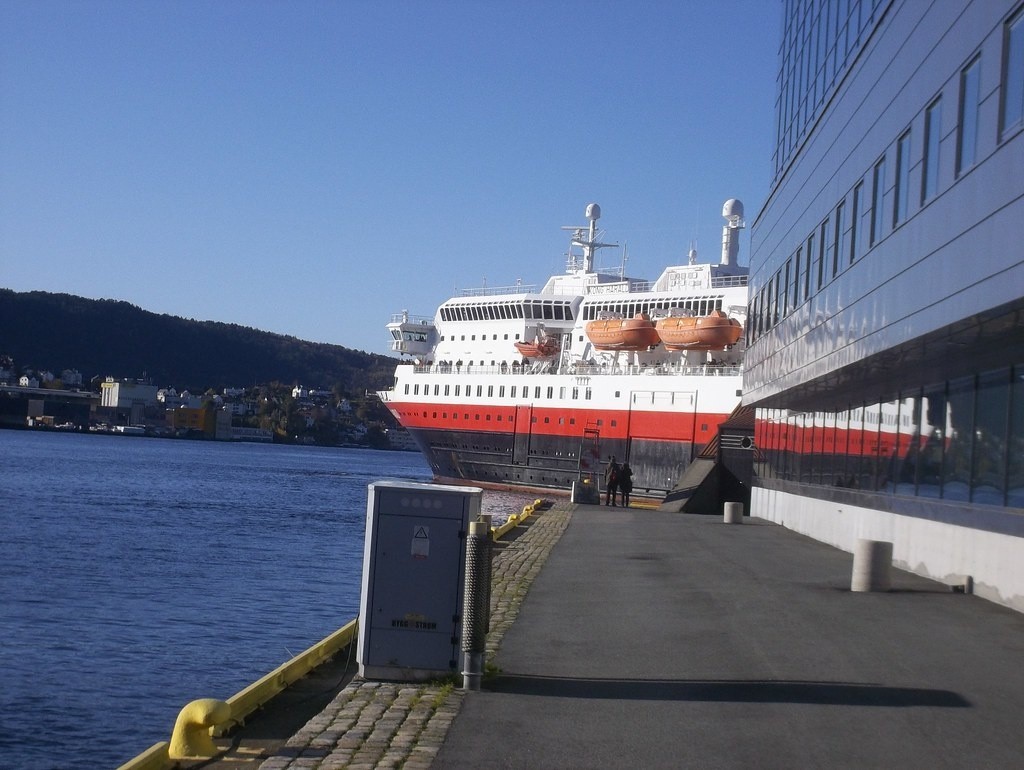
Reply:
[577,420,601,492]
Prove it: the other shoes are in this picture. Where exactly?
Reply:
[605,501,610,506]
[612,502,616,506]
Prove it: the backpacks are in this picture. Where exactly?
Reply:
[610,470,616,481]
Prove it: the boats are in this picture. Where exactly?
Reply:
[374,200,748,503]
[514,341,561,358]
[586,313,661,350]
[654,310,743,352]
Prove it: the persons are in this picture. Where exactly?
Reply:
[399,356,530,374]
[603,455,620,506]
[618,462,633,508]
[587,359,736,375]
